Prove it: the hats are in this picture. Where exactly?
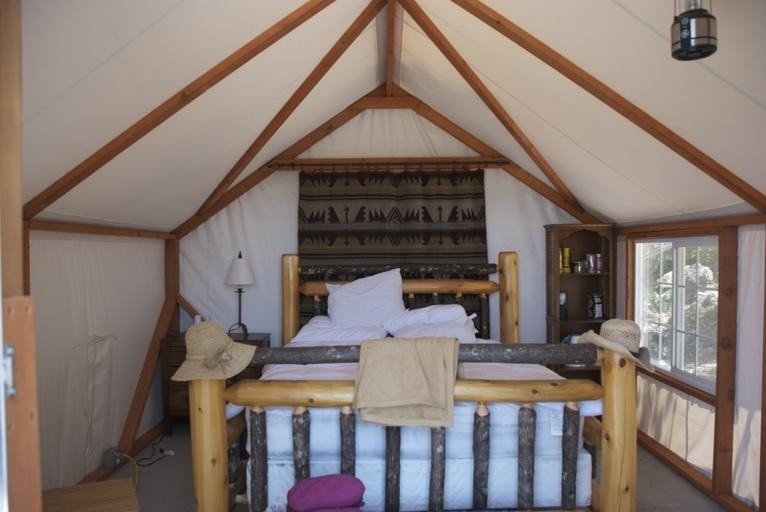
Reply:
[171,321,257,380]
[287,474,365,510]
[579,318,655,373]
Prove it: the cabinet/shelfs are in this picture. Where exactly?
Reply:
[542,222,617,370]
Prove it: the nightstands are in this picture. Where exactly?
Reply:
[554,367,603,423]
[159,333,271,419]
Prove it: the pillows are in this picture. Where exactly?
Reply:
[409,303,467,324]
[324,267,406,327]
[534,397,602,416]
[381,308,420,336]
[395,318,477,344]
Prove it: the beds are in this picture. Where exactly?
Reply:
[187,251,636,512]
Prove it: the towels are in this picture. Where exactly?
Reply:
[351,336,461,428]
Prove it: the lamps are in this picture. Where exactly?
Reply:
[224,251,255,336]
[670,0,718,61]
